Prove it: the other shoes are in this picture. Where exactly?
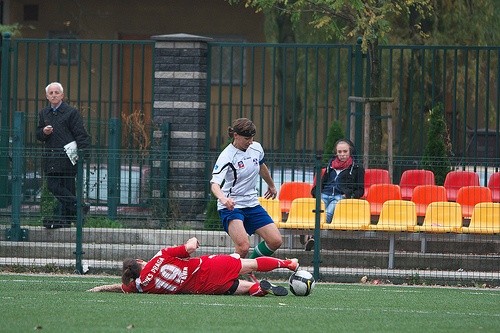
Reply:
[74,203,90,227]
[305,239,314,251]
[300,234,308,244]
[43,215,71,228]
[240,272,257,282]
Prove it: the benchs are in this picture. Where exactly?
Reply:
[258,169,500,269]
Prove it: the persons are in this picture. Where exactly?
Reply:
[209,118,283,284]
[34,82,90,229]
[299,138,365,251]
[85,237,300,296]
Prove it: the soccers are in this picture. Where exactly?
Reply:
[288,270,316,296]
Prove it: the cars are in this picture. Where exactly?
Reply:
[256,168,318,199]
[0,161,152,215]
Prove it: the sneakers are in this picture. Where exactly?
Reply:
[260,280,288,296]
[287,257,299,272]
